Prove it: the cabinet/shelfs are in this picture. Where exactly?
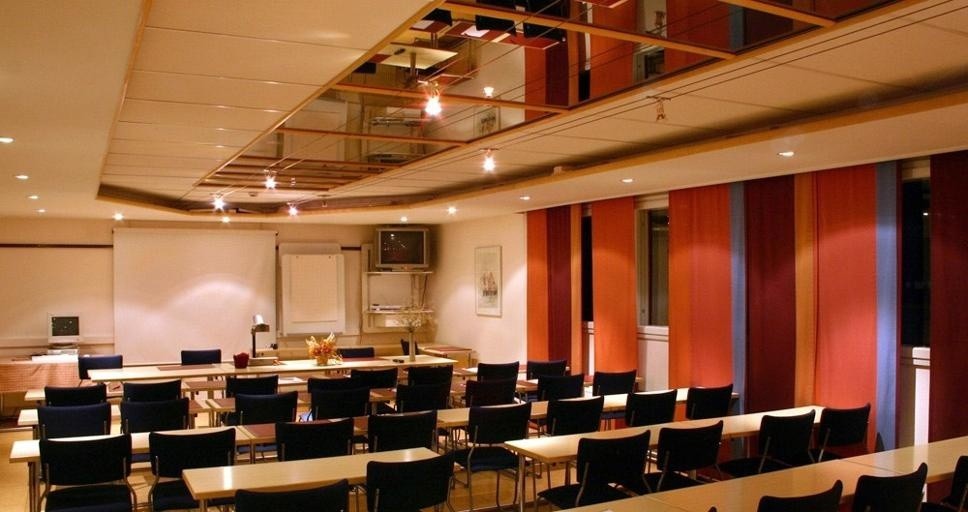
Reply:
[363,270,434,315]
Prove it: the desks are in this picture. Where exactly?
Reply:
[0,356,80,421]
[9,381,250,512]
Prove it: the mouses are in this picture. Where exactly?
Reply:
[84,354,90,357]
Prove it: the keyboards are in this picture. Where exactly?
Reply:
[31,354,79,362]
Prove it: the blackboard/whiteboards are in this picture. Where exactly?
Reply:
[0,243,363,350]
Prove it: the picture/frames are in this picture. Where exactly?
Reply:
[474,245,503,317]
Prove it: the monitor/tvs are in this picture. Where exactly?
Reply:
[374,227,430,271]
[47,315,81,349]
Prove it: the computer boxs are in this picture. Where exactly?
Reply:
[48,347,80,354]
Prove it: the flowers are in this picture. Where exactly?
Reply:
[306,333,342,363]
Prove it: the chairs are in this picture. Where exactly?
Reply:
[37,353,968,512]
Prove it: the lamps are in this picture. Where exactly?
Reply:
[250,313,269,357]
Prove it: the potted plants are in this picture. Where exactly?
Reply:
[394,296,433,363]
